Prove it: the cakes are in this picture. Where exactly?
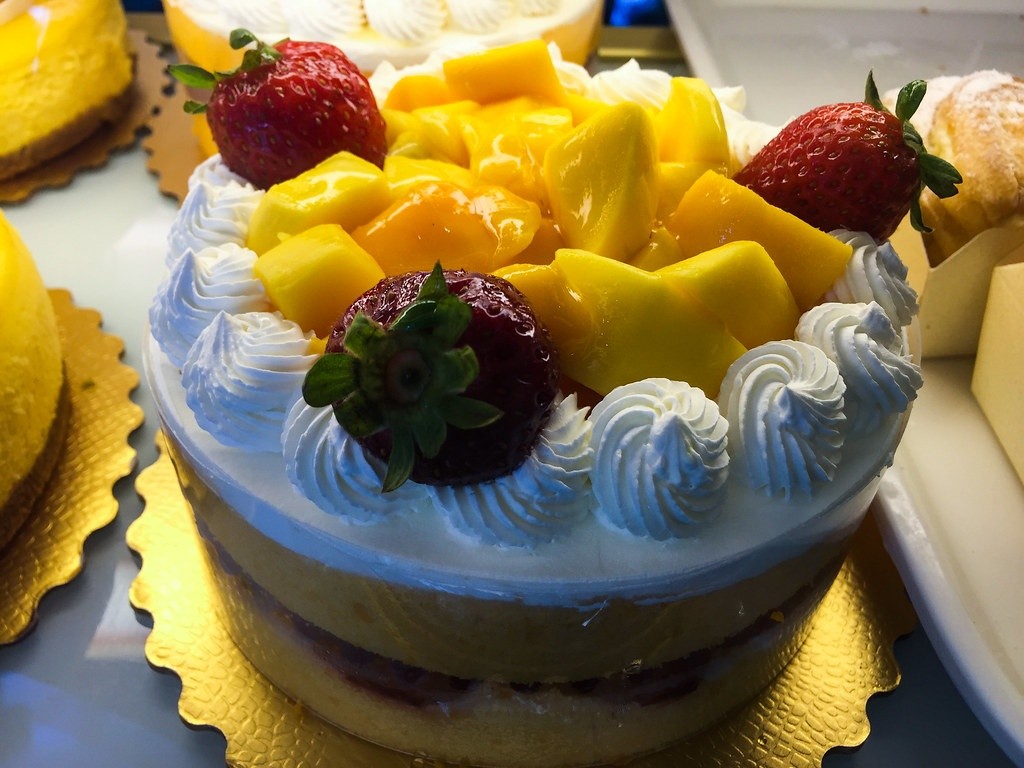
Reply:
[0,0,966,768]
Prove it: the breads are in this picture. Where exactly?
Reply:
[872,71,1023,265]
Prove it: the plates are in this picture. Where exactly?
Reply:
[664,1,1024,767]
